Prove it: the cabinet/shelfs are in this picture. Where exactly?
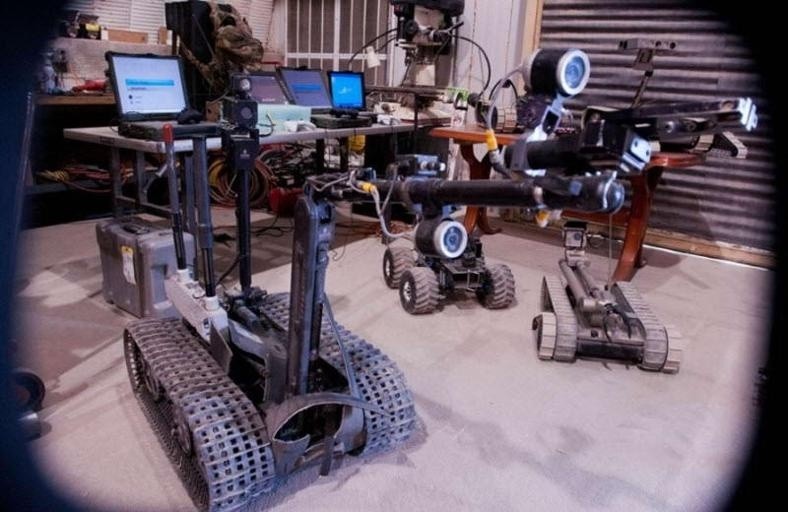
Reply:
[30,94,116,226]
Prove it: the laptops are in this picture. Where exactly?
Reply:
[327,70,387,123]
[104,51,218,140]
[233,70,295,105]
[275,67,373,129]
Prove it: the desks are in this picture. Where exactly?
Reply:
[430,126,707,284]
[62,118,442,280]
